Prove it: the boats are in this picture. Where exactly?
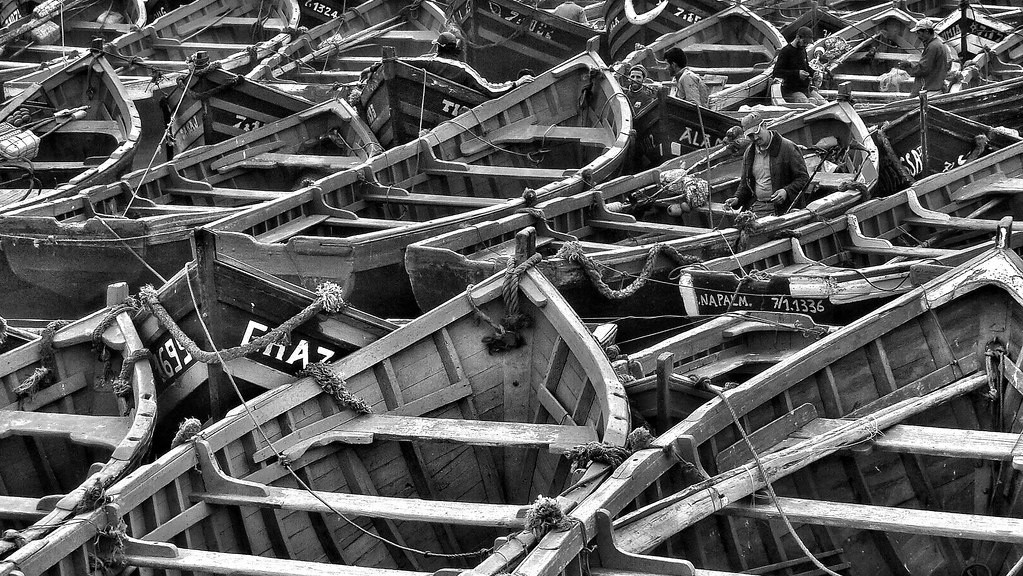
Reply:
[0,0,1023,576]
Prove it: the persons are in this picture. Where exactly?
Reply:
[551,0,588,23]
[722,113,810,219]
[772,25,830,105]
[898,20,952,98]
[664,48,708,106]
[626,63,649,115]
[360,32,535,98]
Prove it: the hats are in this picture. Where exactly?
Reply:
[910,17,933,33]
[797,26,814,45]
[628,65,648,79]
[741,112,764,139]
[437,33,456,47]
